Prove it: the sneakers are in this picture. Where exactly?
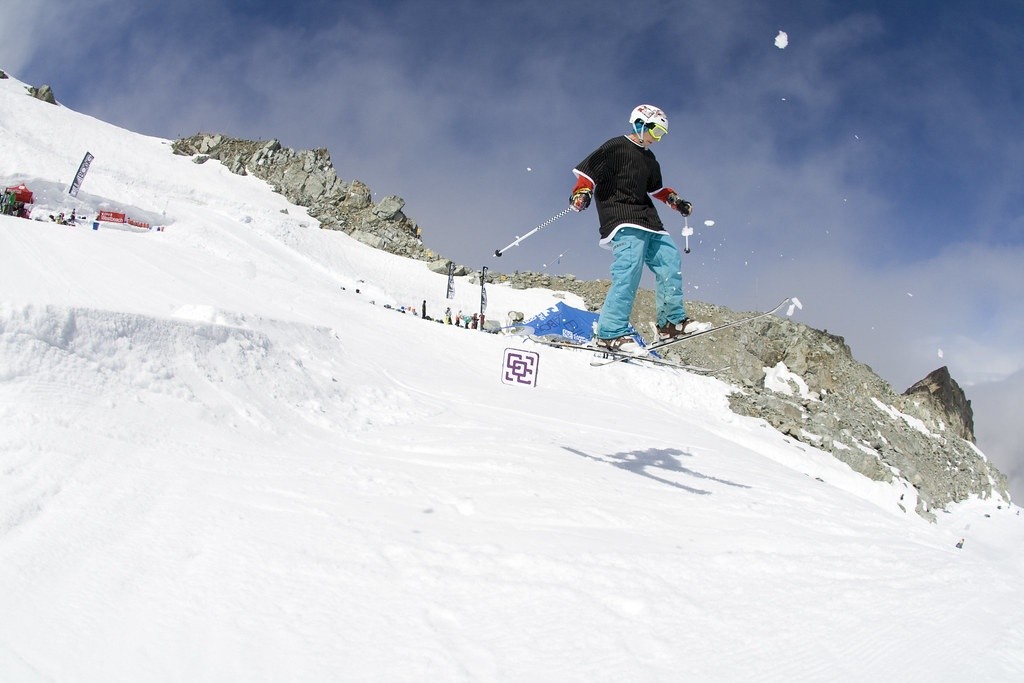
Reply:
[599,334,648,357]
[657,318,712,343]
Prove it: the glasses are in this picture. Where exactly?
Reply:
[649,124,667,142]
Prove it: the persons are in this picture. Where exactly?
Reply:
[569,103,717,360]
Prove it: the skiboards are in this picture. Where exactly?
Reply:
[589,297,793,370]
[526,334,731,374]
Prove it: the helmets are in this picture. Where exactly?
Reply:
[629,105,669,131]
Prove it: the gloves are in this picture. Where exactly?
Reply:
[569,194,589,213]
[677,199,693,216]
